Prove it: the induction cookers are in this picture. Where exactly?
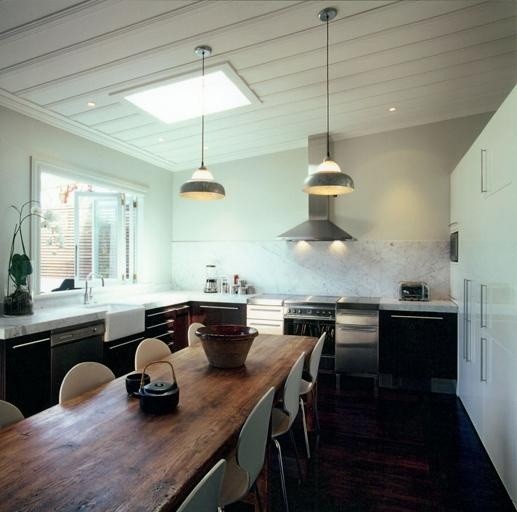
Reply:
[284,295,341,319]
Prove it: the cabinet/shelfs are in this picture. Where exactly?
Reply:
[246,303,284,334]
[0,318,50,415]
[146,296,188,354]
[189,297,246,333]
[379,302,458,396]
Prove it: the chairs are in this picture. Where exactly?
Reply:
[271,351,310,512]
[176,457,226,511]
[135,338,171,369]
[57,362,115,402]
[0,400,25,427]
[219,385,276,512]
[188,322,206,346]
[299,331,327,431]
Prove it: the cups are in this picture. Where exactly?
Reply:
[126,373,151,394]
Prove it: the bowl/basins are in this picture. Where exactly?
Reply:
[195,325,259,368]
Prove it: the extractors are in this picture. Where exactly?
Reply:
[276,133,359,241]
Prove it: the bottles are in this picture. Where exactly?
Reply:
[221,274,255,295]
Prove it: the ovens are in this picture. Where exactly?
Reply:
[284,318,336,375]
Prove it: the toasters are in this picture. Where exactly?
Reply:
[399,282,430,300]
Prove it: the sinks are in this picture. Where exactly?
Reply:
[84,272,105,303]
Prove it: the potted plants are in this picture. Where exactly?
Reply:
[2,201,48,316]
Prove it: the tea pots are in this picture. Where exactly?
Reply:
[131,361,180,417]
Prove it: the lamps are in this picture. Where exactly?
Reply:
[302,7,354,195]
[179,44,225,200]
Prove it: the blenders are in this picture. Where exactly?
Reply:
[203,265,219,293]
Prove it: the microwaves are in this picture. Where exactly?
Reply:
[449,232,458,262]
